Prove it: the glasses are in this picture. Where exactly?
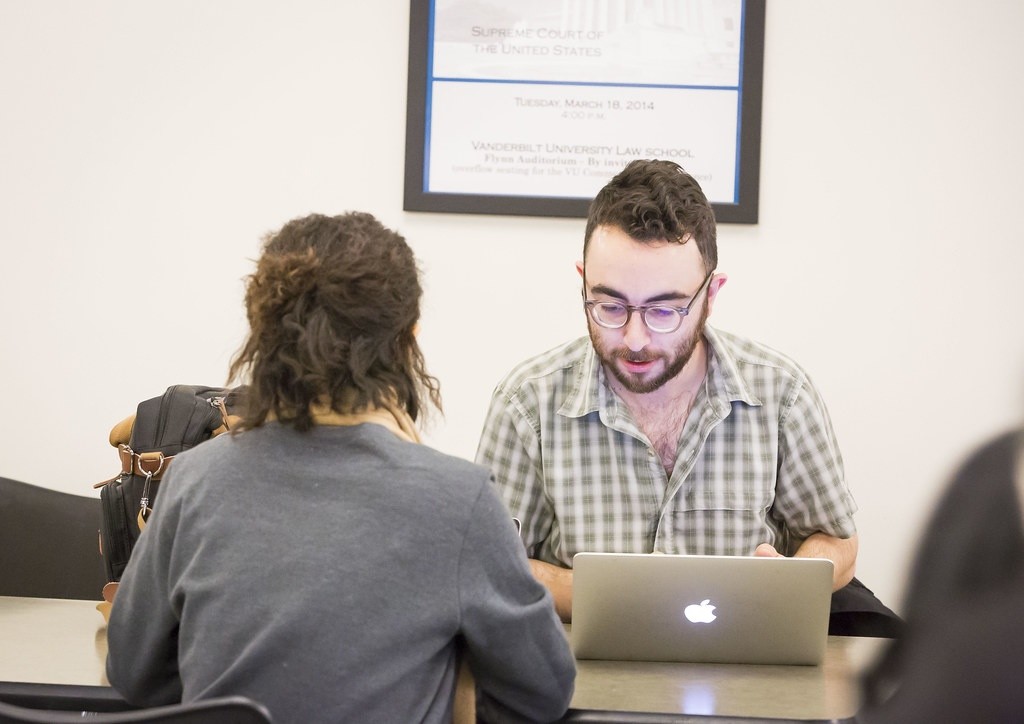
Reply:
[583,270,715,333]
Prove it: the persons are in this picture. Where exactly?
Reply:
[471,159,859,625]
[104,209,576,724]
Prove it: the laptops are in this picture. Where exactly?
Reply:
[569,553,835,665]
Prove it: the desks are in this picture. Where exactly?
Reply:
[0,595,895,724]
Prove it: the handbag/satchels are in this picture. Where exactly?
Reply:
[94,385,249,580]
